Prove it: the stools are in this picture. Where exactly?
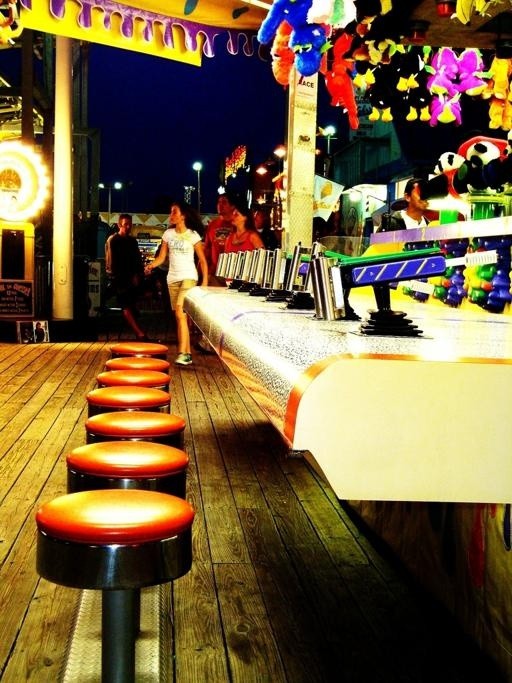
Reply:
[67,441,191,499]
[86,409,186,446]
[84,384,171,415]
[97,368,171,388]
[109,341,169,358]
[104,356,171,371]
[35,488,195,683]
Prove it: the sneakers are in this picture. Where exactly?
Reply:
[187,354,193,364]
[175,353,188,365]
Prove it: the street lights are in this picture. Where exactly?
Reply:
[98,181,122,225]
[193,162,203,213]
[318,125,337,156]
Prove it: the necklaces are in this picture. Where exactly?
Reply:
[233,228,247,248]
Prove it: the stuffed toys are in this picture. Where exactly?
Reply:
[358,11,425,38]
[438,0,501,30]
[369,41,396,123]
[489,96,512,128]
[460,49,488,96]
[305,1,356,28]
[425,48,463,128]
[456,134,512,193]
[257,1,326,78]
[358,2,408,19]
[492,51,507,101]
[321,29,361,133]
[268,19,292,87]
[416,152,466,199]
[399,43,432,122]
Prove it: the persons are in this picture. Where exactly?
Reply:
[196,193,237,287]
[104,212,149,342]
[376,178,432,232]
[142,202,209,367]
[255,205,278,249]
[223,205,266,254]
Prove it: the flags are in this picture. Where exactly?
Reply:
[314,175,346,221]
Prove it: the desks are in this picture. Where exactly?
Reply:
[180,279,512,638]
